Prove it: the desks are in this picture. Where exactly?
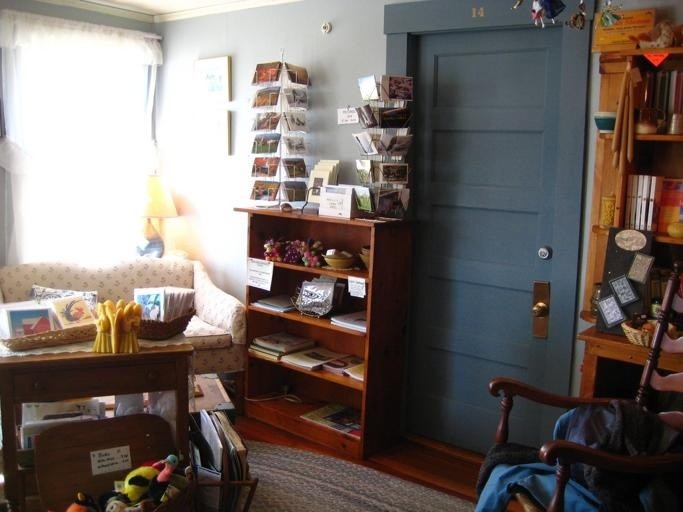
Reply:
[0,345,195,512]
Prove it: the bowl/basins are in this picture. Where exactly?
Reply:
[321,253,353,269]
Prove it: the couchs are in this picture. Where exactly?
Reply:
[0,257,248,416]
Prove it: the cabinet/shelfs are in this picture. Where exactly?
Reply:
[233,207,417,462]
[576,47,683,399]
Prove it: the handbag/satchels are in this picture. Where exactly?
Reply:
[554,397,683,511]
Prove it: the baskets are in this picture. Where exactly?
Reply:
[621,318,654,348]
[140,307,197,339]
[0,322,96,351]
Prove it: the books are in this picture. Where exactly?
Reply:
[636,68,682,134]
[298,399,362,440]
[245,329,365,383]
[620,171,682,234]
[196,405,252,510]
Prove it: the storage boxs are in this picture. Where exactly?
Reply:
[35,414,198,512]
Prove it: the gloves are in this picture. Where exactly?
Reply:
[93,299,142,353]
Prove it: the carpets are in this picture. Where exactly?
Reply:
[235,438,479,512]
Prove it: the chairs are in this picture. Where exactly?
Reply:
[489,262,683,512]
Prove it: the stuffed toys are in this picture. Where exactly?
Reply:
[60,451,181,511]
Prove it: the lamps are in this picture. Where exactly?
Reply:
[146,170,179,237]
